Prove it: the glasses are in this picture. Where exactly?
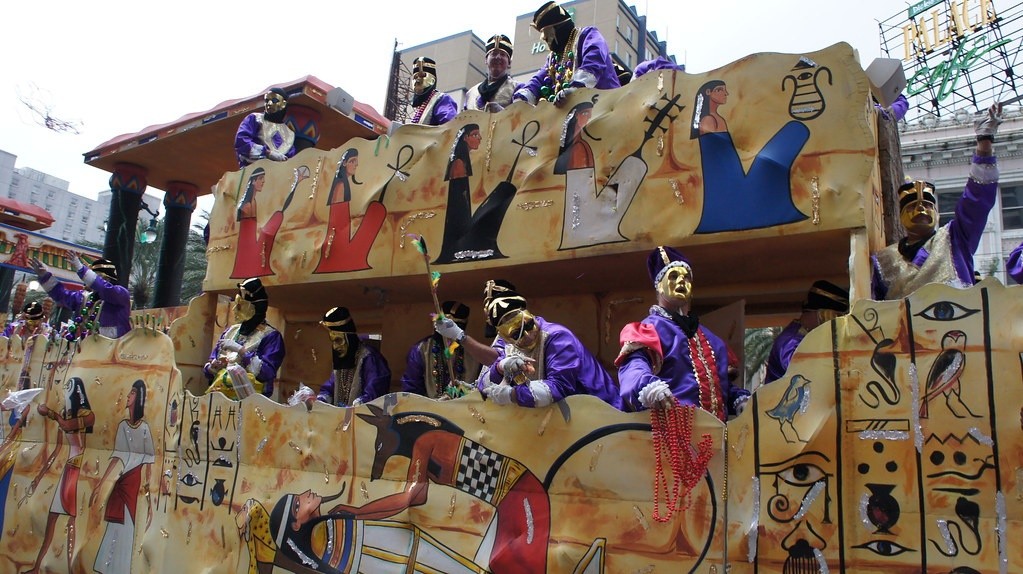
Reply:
[510,318,534,340]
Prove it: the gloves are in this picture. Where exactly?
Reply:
[552,89,565,105]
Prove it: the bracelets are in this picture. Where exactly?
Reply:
[977,134,995,142]
[456,332,468,344]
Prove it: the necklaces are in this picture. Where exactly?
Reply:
[411,90,435,123]
[650,397,713,523]
[431,340,465,397]
[541,27,578,102]
[722,427,727,501]
[65,291,104,341]
[235,326,261,345]
[337,343,362,407]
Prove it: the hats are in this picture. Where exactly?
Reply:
[898,181,937,217]
[237,277,268,303]
[646,246,692,290]
[529,1,571,32]
[485,34,513,59]
[89,259,119,281]
[803,279,849,313]
[442,300,470,331]
[324,306,355,333]
[413,57,437,77]
[483,280,525,326]
[24,302,45,319]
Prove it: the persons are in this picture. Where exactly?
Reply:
[512,0,622,106]
[411,57,458,126]
[404,246,850,422]
[41,296,55,323]
[203,277,285,401]
[462,34,516,113]
[233,87,296,170]
[871,89,1023,302]
[314,305,394,409]
[28,250,133,341]
[3,302,62,343]
